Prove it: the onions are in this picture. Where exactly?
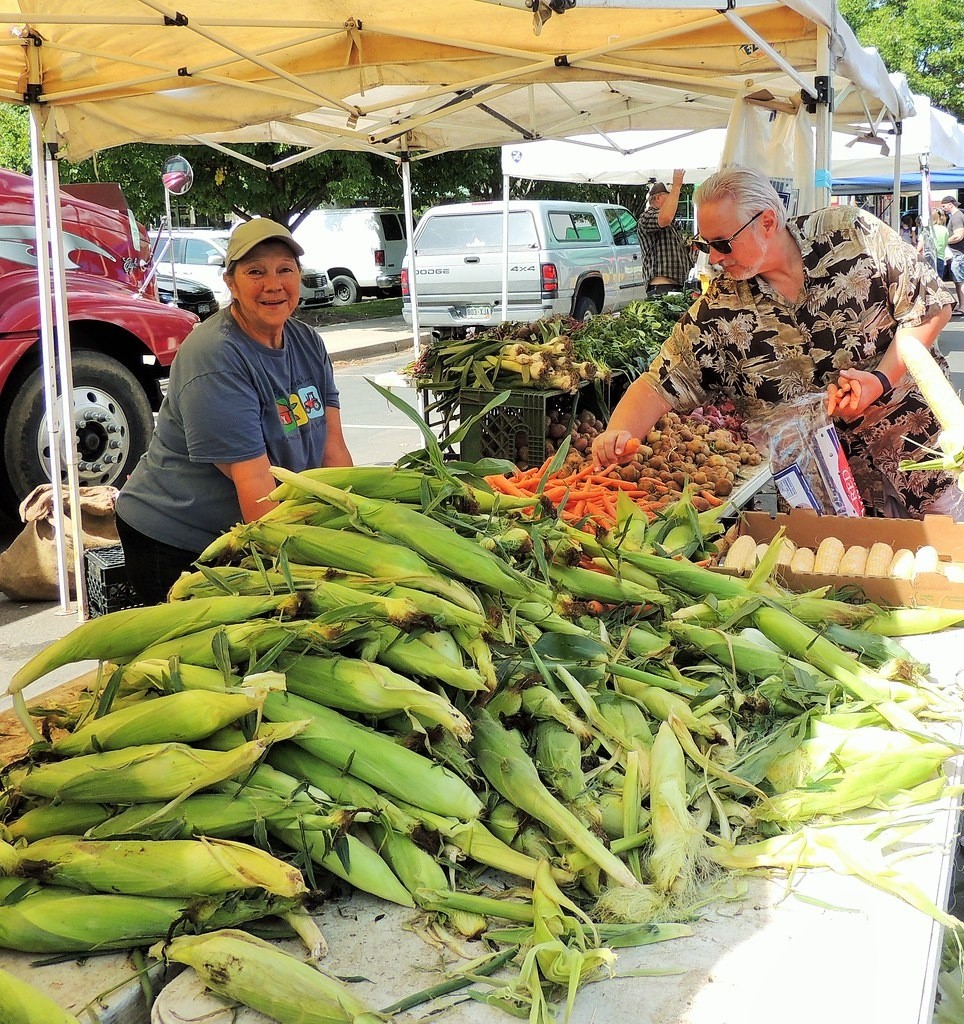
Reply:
[682,399,749,444]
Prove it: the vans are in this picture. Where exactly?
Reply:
[225,207,418,306]
[400,199,647,347]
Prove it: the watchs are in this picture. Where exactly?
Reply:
[870,371,895,405]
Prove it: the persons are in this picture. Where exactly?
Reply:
[592,166,964,523]
[637,169,698,301]
[115,218,354,606]
[900,196,964,317]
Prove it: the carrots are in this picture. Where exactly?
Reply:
[482,458,722,618]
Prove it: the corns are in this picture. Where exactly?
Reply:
[0,467,964,1024]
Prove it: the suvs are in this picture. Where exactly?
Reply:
[0,161,202,534]
[143,226,336,314]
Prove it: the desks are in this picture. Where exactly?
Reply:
[1,432,964,1024]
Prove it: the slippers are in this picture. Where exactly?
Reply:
[952,309,964,316]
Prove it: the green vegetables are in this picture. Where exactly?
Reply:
[566,290,697,373]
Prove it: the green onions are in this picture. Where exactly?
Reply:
[409,337,608,393]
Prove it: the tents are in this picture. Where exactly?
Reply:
[501,95,964,324]
[0,0,917,623]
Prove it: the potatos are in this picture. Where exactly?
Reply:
[516,411,761,494]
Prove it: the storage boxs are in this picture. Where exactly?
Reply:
[459,369,633,466]
[712,513,964,610]
[84,543,142,619]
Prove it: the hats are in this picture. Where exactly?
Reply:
[225,217,305,269]
[649,182,682,195]
[941,196,961,206]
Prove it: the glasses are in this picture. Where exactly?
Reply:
[690,209,767,254]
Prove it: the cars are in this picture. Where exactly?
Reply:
[149,268,220,324]
